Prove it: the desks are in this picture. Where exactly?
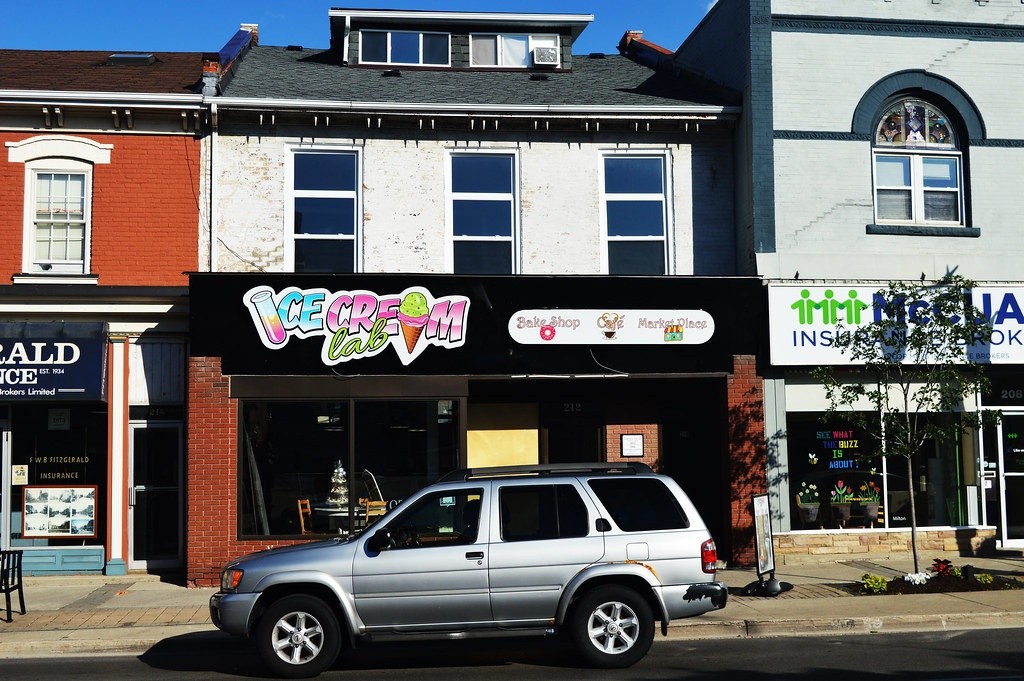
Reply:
[843,498,872,529]
[315,507,381,534]
[328,513,384,534]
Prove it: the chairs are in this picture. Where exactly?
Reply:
[365,501,388,527]
[297,499,326,535]
[855,505,884,528]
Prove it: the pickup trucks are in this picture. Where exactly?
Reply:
[208,461,728,679]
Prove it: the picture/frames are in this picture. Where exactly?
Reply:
[21,485,97,539]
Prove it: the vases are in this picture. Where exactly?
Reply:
[859,501,880,521]
[795,494,820,522]
[830,502,852,520]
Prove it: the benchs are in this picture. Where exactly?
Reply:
[0,549,27,623]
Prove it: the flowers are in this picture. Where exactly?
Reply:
[830,480,853,503]
[798,482,818,504]
[858,481,880,503]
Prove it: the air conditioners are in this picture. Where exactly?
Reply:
[534,46,559,67]
